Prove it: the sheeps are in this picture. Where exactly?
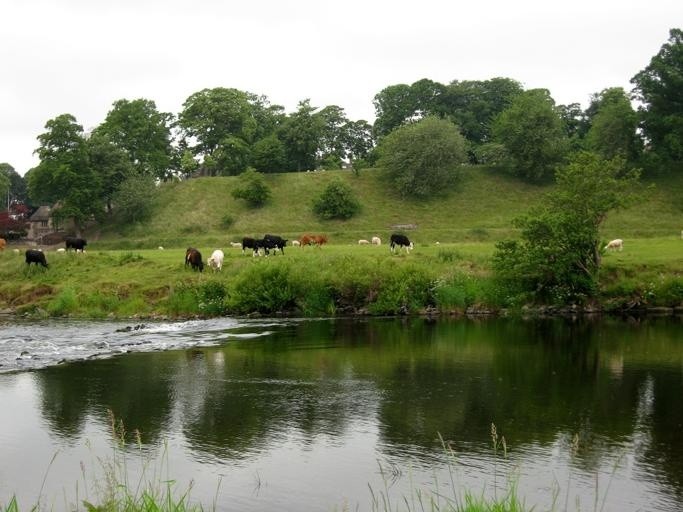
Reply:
[359,236,382,245]
[230,242,242,248]
[292,240,299,246]
[158,246,164,251]
[605,239,625,252]
[57,248,65,253]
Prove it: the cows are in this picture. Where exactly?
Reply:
[241,232,288,257]
[24,249,49,269]
[65,238,87,252]
[299,234,327,249]
[184,246,225,272]
[389,233,414,254]
